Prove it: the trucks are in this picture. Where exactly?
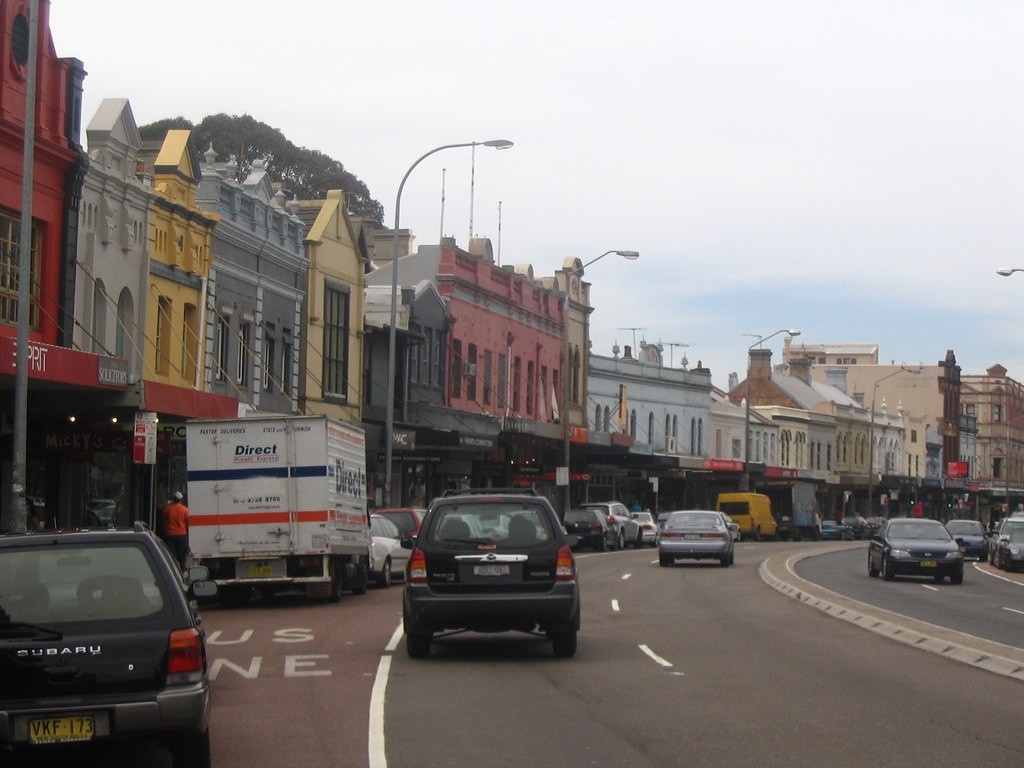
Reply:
[769,481,821,542]
[185,414,371,608]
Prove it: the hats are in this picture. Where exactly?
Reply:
[173,492,183,499]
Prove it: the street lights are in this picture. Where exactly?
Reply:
[745,329,801,491]
[564,250,639,511]
[383,140,514,508]
[868,369,921,515]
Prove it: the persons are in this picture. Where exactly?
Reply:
[160,491,189,575]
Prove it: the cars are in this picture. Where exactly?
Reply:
[659,510,736,568]
[373,508,429,543]
[867,517,964,584]
[632,511,675,546]
[943,519,988,561]
[819,515,887,539]
[369,515,409,588]
[988,510,1024,572]
[561,510,618,554]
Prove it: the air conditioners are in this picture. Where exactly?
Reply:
[463,363,476,375]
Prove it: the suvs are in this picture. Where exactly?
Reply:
[402,486,581,657]
[0,520,219,768]
[579,502,643,549]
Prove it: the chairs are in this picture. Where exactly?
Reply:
[3,582,50,622]
[440,518,471,541]
[77,573,151,618]
[508,515,538,543]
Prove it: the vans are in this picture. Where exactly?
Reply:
[717,492,778,542]
